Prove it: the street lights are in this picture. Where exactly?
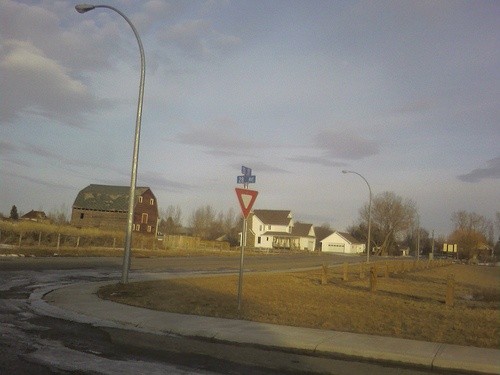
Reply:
[72,2,146,287]
[342,170,374,260]
